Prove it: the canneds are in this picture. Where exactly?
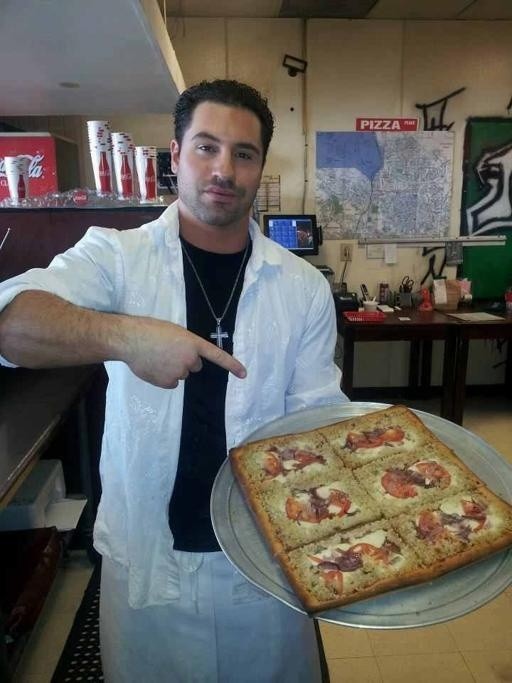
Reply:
[380,283,389,305]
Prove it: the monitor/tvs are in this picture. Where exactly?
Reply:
[263,214,319,256]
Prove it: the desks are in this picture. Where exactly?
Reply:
[334,299,511,428]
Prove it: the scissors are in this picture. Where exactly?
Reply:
[402,275,414,287]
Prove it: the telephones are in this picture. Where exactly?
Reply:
[360,284,370,300]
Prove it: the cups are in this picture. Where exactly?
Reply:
[363,301,378,312]
[4,156,29,198]
[87,120,157,203]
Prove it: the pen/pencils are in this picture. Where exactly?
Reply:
[380,283,389,304]
[400,285,412,292]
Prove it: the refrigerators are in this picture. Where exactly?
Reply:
[0,132,80,202]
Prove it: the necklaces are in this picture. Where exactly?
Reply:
[182,243,249,350]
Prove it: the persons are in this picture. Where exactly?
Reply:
[0,79,351,683]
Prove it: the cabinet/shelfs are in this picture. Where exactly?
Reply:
[1,364,107,679]
